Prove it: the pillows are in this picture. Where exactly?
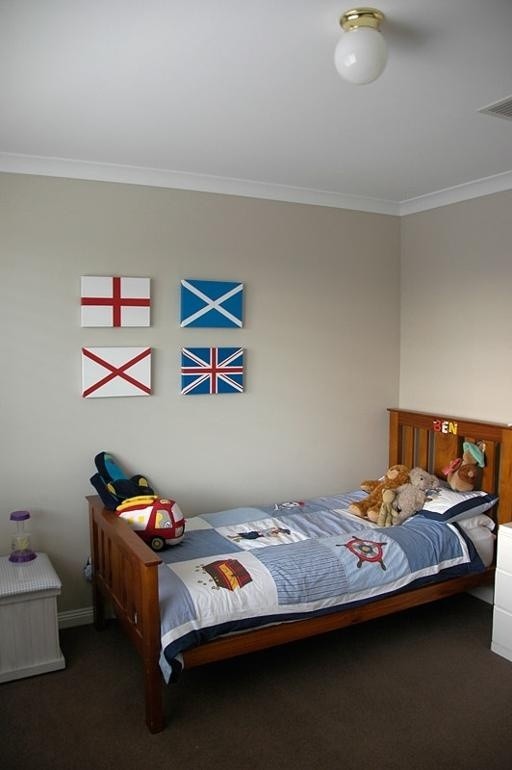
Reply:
[416,479,499,524]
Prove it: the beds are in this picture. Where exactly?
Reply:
[86,409,512,733]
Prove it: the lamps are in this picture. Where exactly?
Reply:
[333,7,387,87]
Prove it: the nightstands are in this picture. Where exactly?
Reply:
[0,552,66,683]
[490,522,512,662]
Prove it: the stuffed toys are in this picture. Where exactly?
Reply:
[389,465,440,526]
[446,438,486,493]
[348,465,411,523]
[377,486,399,527]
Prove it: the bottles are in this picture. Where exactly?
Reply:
[9,510,38,562]
